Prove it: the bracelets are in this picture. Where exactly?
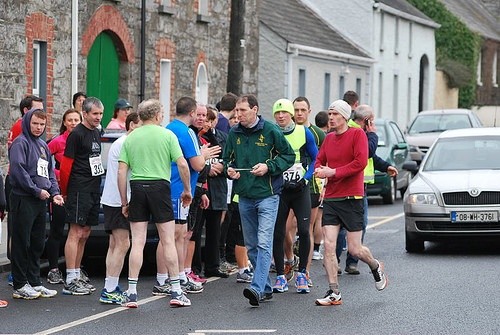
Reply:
[121,203,128,207]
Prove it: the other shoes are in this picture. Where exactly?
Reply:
[0,300,8,307]
[204,269,228,279]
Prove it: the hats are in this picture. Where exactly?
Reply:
[114,99,133,110]
[328,100,351,121]
[272,99,294,118]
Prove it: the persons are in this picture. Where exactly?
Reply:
[271,96,319,293]
[285,91,398,286]
[222,94,297,305]
[185,93,253,283]
[62,97,104,295]
[73,92,103,134]
[46,109,82,284]
[5,109,64,299]
[115,99,193,307]
[106,98,132,130]
[8,95,44,287]
[314,100,388,306]
[151,97,221,296]
[0,175,8,308]
[100,113,143,305]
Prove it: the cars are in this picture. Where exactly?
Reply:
[406,109,484,175]
[358,120,415,203]
[403,128,500,251]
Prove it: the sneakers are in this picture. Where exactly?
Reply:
[99,286,123,304]
[47,270,64,284]
[152,281,186,296]
[13,283,41,300]
[169,291,191,307]
[33,285,57,297]
[218,262,237,275]
[180,277,203,293]
[79,279,96,291]
[237,250,387,306]
[121,292,138,308]
[62,279,91,295]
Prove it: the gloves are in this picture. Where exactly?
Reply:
[283,178,307,193]
